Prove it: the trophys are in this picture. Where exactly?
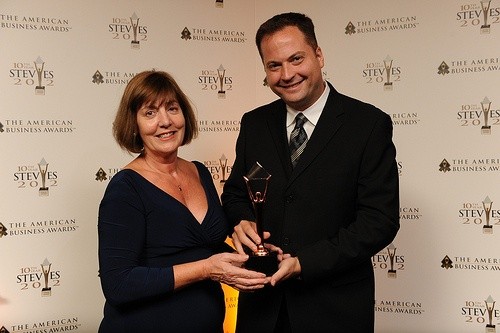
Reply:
[242,161,280,277]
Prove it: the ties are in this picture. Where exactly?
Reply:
[288,112,309,169]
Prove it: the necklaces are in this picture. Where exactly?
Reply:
[144,154,183,191]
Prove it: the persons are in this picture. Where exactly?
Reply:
[220,12,400,333]
[97,65,291,333]
